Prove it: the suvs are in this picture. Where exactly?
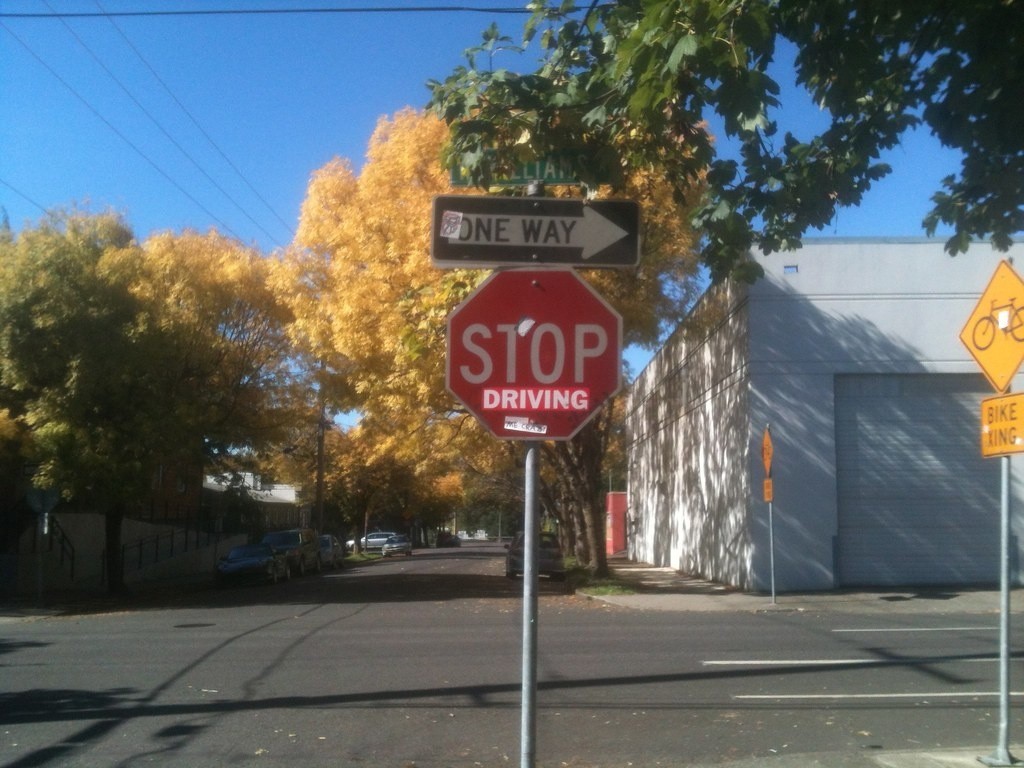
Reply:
[262,528,323,577]
[346,532,399,551]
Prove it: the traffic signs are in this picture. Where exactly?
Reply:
[429,191,644,264]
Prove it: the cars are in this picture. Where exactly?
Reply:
[382,535,412,558]
[316,534,345,571]
[218,543,292,590]
[504,529,567,582]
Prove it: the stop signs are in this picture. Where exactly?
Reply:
[446,266,622,442]
